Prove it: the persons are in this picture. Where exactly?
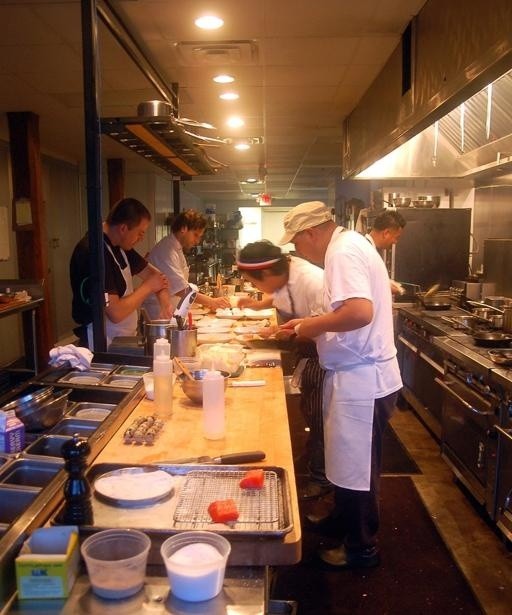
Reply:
[235,237,339,522]
[275,199,407,565]
[138,208,235,320]
[68,197,176,357]
[363,210,406,298]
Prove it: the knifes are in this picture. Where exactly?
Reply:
[150,450,266,466]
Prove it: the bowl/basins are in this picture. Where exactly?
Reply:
[392,196,441,208]
[487,349,512,365]
[0,385,75,433]
[177,370,232,403]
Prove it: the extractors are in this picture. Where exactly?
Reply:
[430,70,512,155]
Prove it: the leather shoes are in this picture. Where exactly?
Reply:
[304,514,339,533]
[320,544,383,575]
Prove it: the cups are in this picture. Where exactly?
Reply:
[212,287,225,298]
[169,327,198,358]
[175,358,200,373]
[159,531,232,603]
[142,371,154,401]
[79,528,151,600]
[223,285,235,296]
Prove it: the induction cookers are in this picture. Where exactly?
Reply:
[447,329,512,371]
[401,306,491,336]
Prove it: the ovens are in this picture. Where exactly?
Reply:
[398,312,444,444]
[433,367,501,521]
[493,381,512,543]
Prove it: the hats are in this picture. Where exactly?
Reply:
[280,202,331,245]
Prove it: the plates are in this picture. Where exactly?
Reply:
[189,306,270,345]
[94,467,176,506]
[70,375,100,385]
[77,407,113,420]
[111,379,138,388]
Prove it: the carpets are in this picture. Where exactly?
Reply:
[286,394,423,475]
[270,477,486,614]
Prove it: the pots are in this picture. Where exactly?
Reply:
[135,100,229,170]
[443,316,512,347]
[142,318,186,365]
[398,293,451,309]
[466,295,512,333]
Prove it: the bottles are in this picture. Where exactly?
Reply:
[152,336,172,360]
[207,216,224,230]
[201,362,226,441]
[230,270,241,293]
[62,435,93,527]
[203,282,210,296]
[152,350,174,417]
